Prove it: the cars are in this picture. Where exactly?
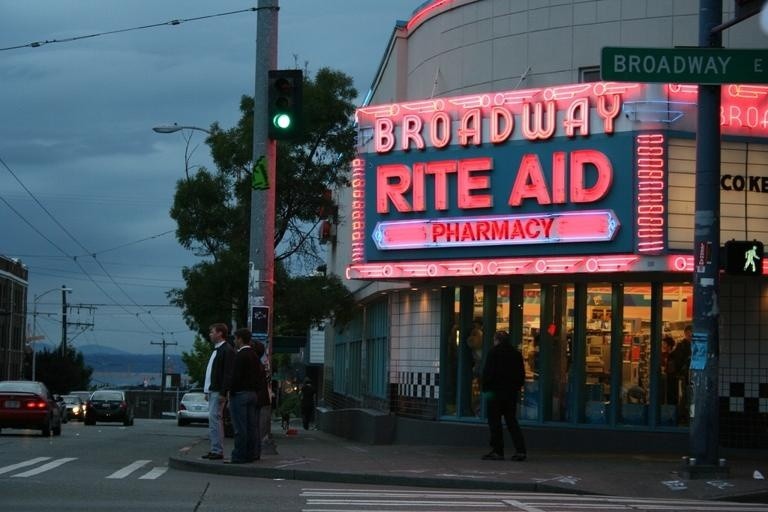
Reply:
[177,387,212,427]
[53,394,67,424]
[85,389,138,428]
[0,380,64,437]
[61,395,86,422]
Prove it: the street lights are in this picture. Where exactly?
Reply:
[149,119,256,179]
[31,284,75,380]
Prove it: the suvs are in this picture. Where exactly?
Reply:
[70,391,93,417]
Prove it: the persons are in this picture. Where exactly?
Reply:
[660,337,679,403]
[479,332,528,462]
[300,377,314,430]
[672,324,692,404]
[202,322,269,465]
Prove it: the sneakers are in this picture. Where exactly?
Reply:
[505,451,532,466]
[480,446,509,463]
[222,455,264,469]
[200,451,226,461]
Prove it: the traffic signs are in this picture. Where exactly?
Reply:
[597,45,767,84]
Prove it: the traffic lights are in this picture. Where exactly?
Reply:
[724,236,768,283]
[263,64,304,141]
